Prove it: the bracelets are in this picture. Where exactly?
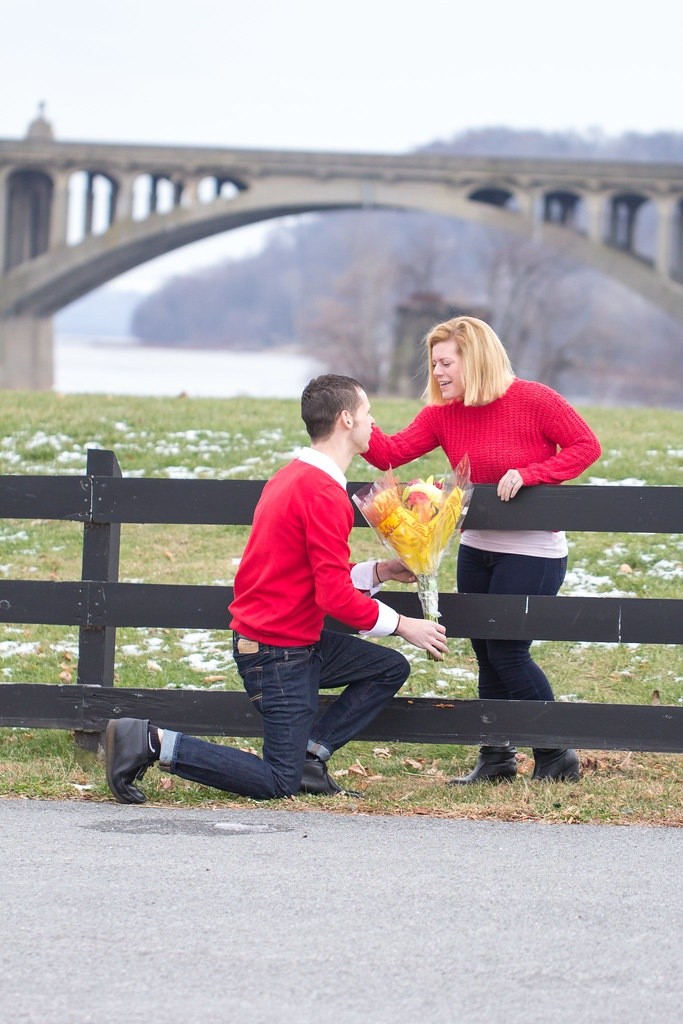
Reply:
[376,562,383,584]
[394,614,402,635]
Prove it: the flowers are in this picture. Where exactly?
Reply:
[355,464,470,662]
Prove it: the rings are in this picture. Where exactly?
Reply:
[508,479,517,485]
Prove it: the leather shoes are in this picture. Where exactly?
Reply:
[105,717,154,805]
[297,760,363,799]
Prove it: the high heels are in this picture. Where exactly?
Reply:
[532,749,580,784]
[449,753,517,785]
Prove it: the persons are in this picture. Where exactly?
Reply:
[358,317,602,785]
[106,375,449,806]
